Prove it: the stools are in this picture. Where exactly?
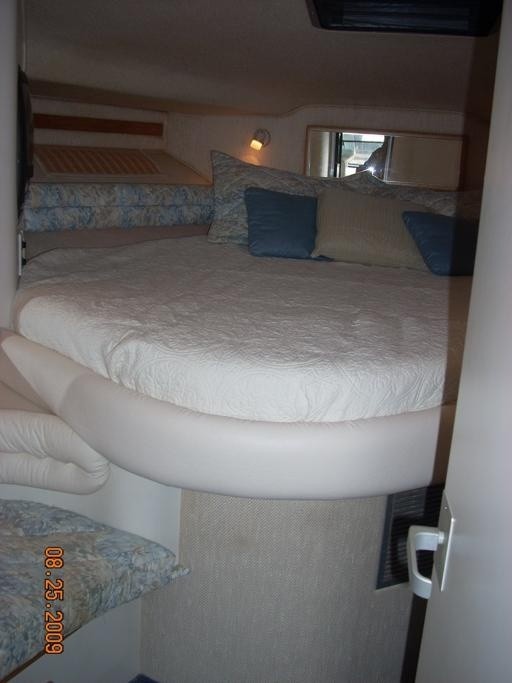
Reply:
[0,409,191,683]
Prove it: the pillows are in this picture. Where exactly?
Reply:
[401,210,478,277]
[244,187,336,263]
[368,175,482,216]
[309,182,436,274]
[204,150,372,245]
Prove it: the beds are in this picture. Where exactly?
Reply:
[0,180,474,683]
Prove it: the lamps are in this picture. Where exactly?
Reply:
[248,128,274,152]
